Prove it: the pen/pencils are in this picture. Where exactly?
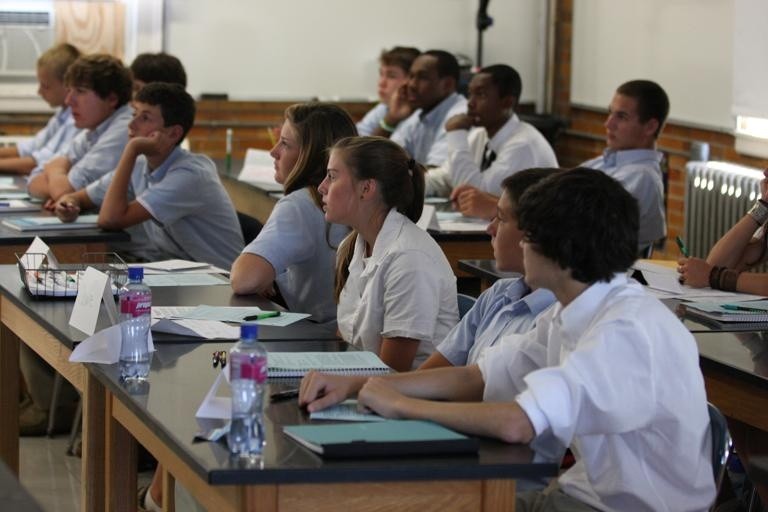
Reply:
[213,351,226,367]
[676,236,690,258]
[243,310,281,321]
[271,389,298,400]
[61,203,69,208]
[721,305,768,311]
[1,198,31,200]
[267,126,276,146]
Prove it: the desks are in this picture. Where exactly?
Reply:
[1,137,766,512]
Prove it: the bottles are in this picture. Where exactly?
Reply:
[229,324,270,457]
[115,264,154,383]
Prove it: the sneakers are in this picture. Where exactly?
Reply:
[19,405,58,436]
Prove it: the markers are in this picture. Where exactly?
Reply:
[34,271,76,289]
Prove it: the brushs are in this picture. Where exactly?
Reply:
[201,94,227,100]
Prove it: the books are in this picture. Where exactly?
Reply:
[283,417,475,458]
[686,314,767,333]
[267,351,389,377]
[680,299,767,322]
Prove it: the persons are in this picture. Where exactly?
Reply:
[677,171,768,294]
[1,43,245,437]
[353,45,559,222]
[448,80,671,249]
[734,331,768,380]
[138,100,360,510]
[300,168,718,512]
[414,168,558,370]
[318,136,460,372]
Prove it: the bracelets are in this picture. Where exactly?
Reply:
[720,268,740,290]
[741,334,766,361]
[708,264,728,290]
[748,199,767,227]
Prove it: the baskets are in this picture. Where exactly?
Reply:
[14,252,129,299]
